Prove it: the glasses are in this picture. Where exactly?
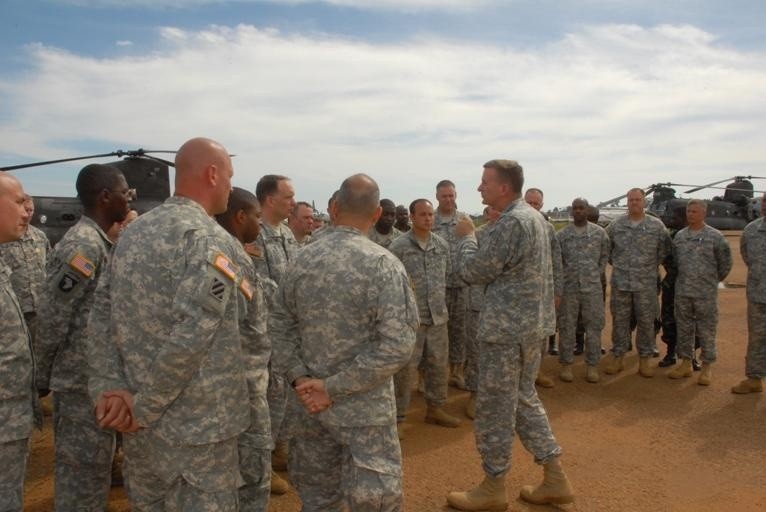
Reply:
[111,187,136,202]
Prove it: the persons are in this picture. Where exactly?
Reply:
[664,198,733,387]
[0,163,421,512]
[657,205,687,369]
[628,268,662,359]
[448,159,577,511]
[389,179,613,439]
[83,136,253,512]
[726,192,766,395]
[603,188,674,378]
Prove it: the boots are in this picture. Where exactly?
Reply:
[111,455,123,487]
[519,462,576,505]
[535,331,714,387]
[271,443,289,468]
[417,365,477,427]
[731,378,763,394]
[270,470,288,495]
[397,422,406,440]
[446,477,508,512]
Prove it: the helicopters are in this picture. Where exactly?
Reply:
[0,143,238,253]
[595,175,765,236]
